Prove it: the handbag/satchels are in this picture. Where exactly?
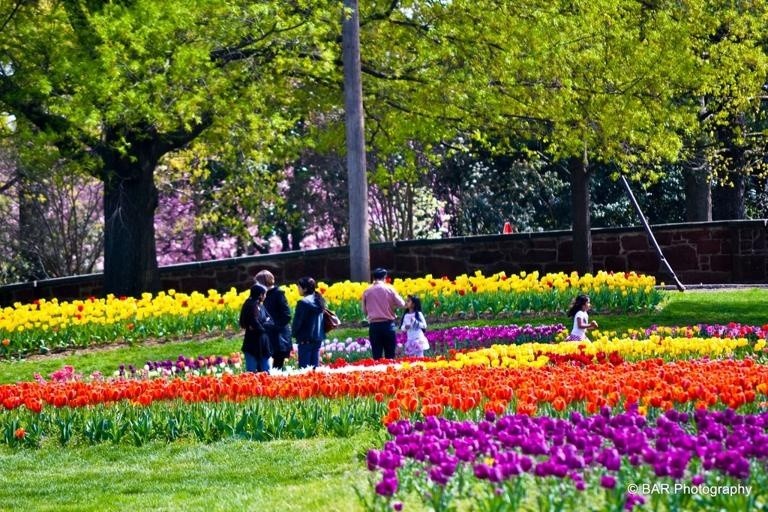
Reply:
[323,307,340,334]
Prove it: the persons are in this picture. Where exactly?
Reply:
[399,294,429,357]
[564,294,598,343]
[254,268,293,369]
[290,277,328,366]
[361,267,405,359]
[241,286,274,370]
